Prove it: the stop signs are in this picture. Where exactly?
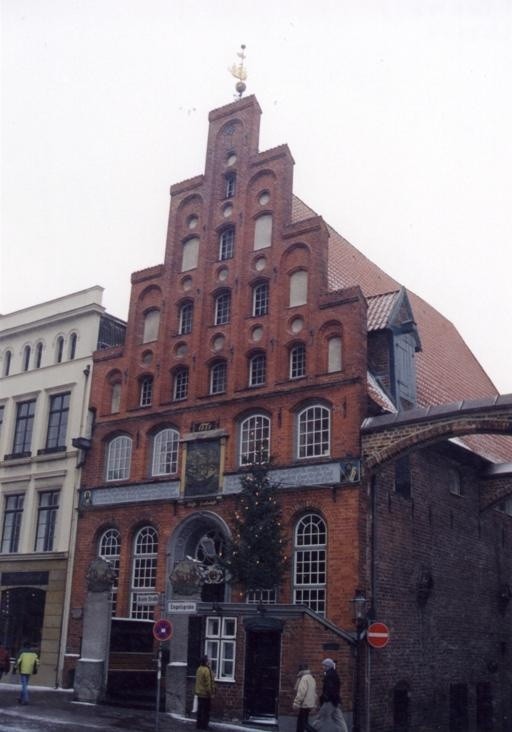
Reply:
[366,622,390,649]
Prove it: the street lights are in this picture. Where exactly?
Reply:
[350,588,369,731]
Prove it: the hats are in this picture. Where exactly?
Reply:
[322,658,334,668]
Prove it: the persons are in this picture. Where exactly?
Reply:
[12,641,40,707]
[291,663,321,732]
[311,658,348,732]
[0,641,10,681]
[194,655,215,732]
